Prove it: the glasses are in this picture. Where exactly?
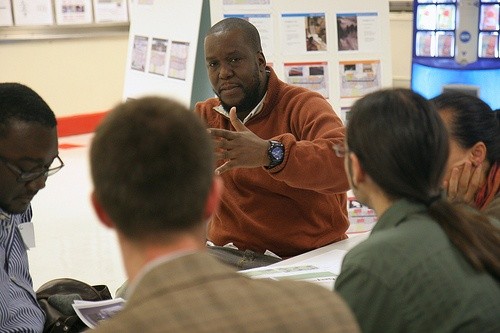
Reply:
[1,152,64,182]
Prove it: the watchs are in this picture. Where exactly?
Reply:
[264,138,285,171]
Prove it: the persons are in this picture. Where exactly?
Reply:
[192,18,351,273]
[82,94,360,332]
[427,92,500,221]
[332,87,500,333]
[1,83,65,333]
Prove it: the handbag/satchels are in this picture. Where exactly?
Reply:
[35,277,112,333]
